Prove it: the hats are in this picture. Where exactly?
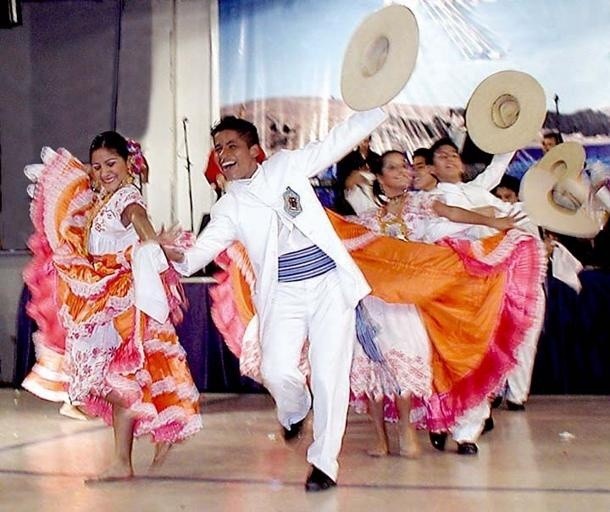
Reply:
[464,69,548,156]
[534,140,587,178]
[339,3,420,112]
[517,164,608,239]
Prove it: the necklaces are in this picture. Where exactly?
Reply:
[390,194,403,206]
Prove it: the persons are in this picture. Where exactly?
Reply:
[205,145,267,202]
[543,132,563,152]
[266,115,298,153]
[160,105,391,494]
[209,137,553,457]
[545,208,610,270]
[24,130,188,485]
[331,133,383,217]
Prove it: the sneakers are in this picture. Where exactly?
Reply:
[280,410,303,445]
[453,440,480,455]
[429,431,446,452]
[304,466,338,492]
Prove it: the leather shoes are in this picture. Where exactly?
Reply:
[491,394,525,411]
